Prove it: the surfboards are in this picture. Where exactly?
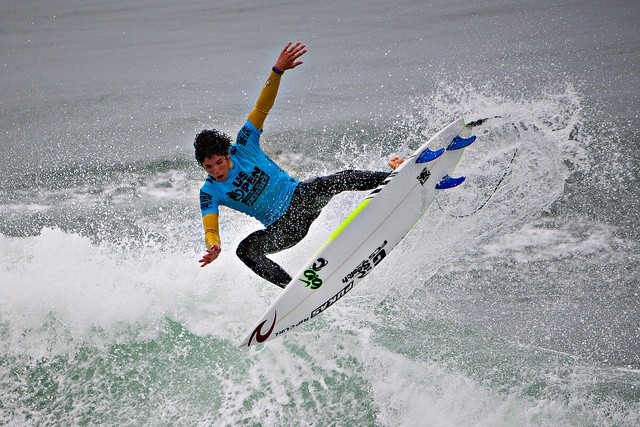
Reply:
[237,117,476,349]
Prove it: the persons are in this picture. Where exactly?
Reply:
[193,41,405,289]
